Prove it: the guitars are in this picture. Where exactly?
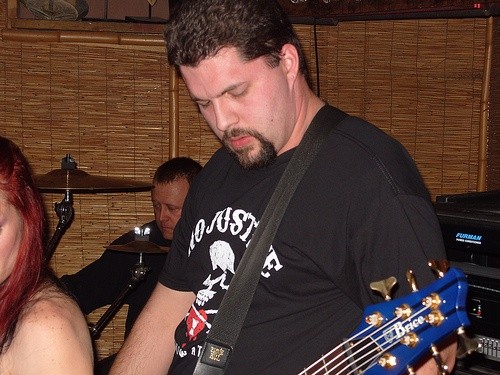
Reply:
[295,258,479,375]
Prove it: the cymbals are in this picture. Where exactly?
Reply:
[102,240,171,253]
[33,169,156,194]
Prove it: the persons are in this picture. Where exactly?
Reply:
[57,156,207,375]
[109,0,457,375]
[1,139,94,375]
